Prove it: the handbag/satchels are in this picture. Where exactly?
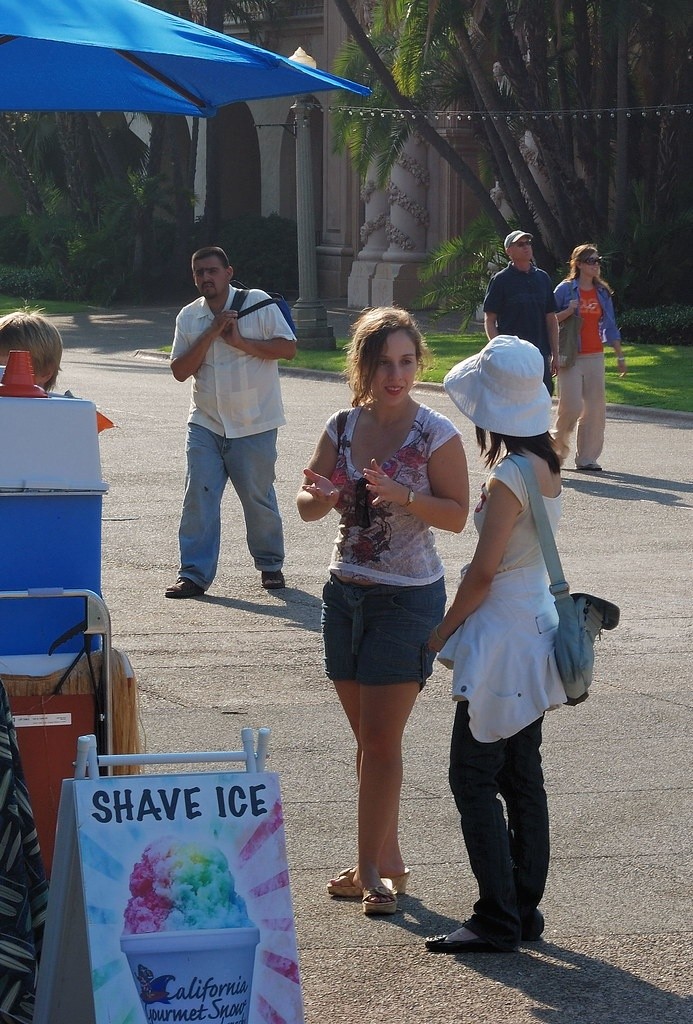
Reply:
[558,280,583,367]
[229,279,296,336]
[555,593,619,706]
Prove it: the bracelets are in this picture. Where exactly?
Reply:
[617,356,624,361]
[435,623,446,642]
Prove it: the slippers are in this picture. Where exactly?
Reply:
[327,867,410,896]
[362,885,398,915]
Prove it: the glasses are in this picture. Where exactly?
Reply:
[509,241,531,248]
[583,258,602,265]
[354,477,371,528]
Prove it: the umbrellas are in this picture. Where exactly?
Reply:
[0,0,373,118]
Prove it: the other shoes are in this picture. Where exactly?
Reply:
[577,464,602,470]
[425,934,500,952]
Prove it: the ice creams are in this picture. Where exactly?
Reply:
[122,833,256,935]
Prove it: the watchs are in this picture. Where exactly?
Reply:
[400,487,415,507]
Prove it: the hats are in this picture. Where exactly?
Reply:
[443,334,552,437]
[504,231,533,247]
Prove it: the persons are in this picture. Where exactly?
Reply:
[554,245,627,470]
[0,311,64,392]
[425,334,568,953]
[298,307,469,914]
[165,248,298,598]
[482,231,559,396]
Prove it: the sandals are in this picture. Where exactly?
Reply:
[165,577,205,597]
[262,570,284,589]
